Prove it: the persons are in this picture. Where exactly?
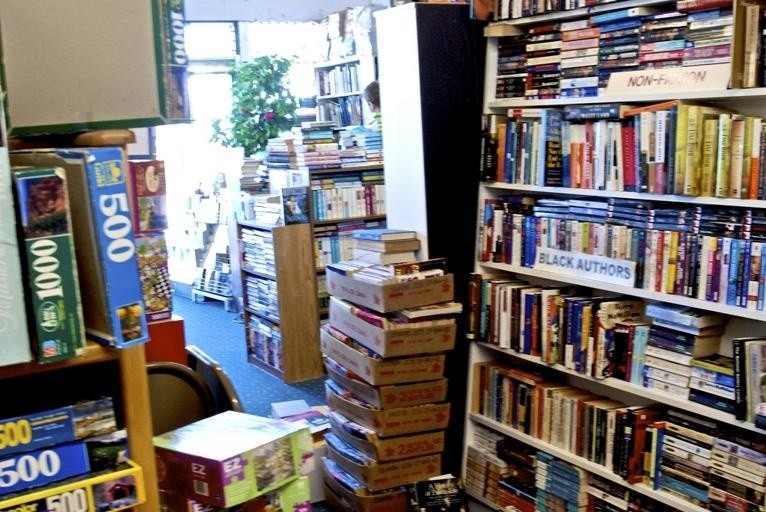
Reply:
[364,82,383,124]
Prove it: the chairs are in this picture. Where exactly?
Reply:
[188,344,243,414]
[120,361,212,444]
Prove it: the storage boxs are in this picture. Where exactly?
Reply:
[319,266,456,512]
[153,411,314,508]
[0,0,191,137]
[158,476,312,512]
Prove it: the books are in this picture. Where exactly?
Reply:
[190,187,234,298]
[318,228,462,510]
[313,62,363,126]
[237,122,387,371]
[270,399,330,449]
[462,1,766,511]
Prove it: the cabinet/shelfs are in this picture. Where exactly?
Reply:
[458,1,766,512]
